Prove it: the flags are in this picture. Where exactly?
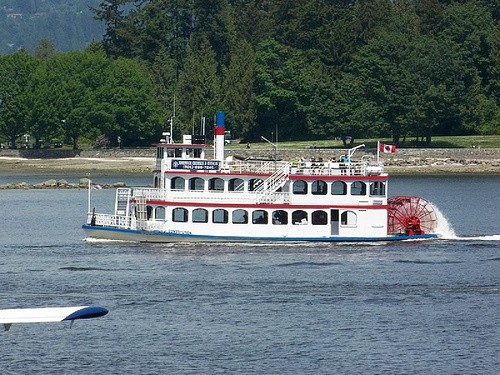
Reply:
[379,143,396,153]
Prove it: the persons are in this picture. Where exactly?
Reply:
[293,153,353,175]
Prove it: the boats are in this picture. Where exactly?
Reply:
[80,104,439,242]
